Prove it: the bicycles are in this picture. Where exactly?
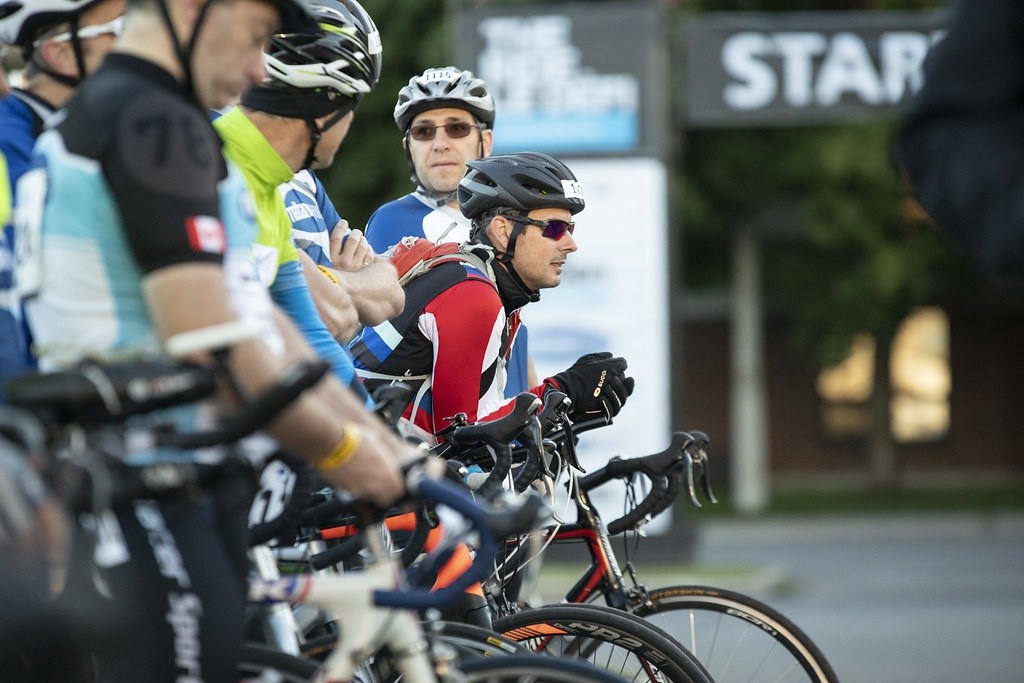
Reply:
[1,319,841,683]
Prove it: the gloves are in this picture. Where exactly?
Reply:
[551,352,634,424]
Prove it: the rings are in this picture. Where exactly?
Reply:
[362,261,370,266]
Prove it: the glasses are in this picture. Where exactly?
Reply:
[502,213,576,241]
[407,121,485,140]
[32,13,126,47]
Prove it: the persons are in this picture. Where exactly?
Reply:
[1,0,636,683]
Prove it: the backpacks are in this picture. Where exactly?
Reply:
[380,236,496,282]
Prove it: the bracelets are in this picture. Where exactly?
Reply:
[313,265,340,287]
[307,424,359,477]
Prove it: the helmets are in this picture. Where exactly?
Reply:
[240,0,383,119]
[259,0,326,35]
[393,66,495,135]
[0,0,99,47]
[456,152,586,219]
